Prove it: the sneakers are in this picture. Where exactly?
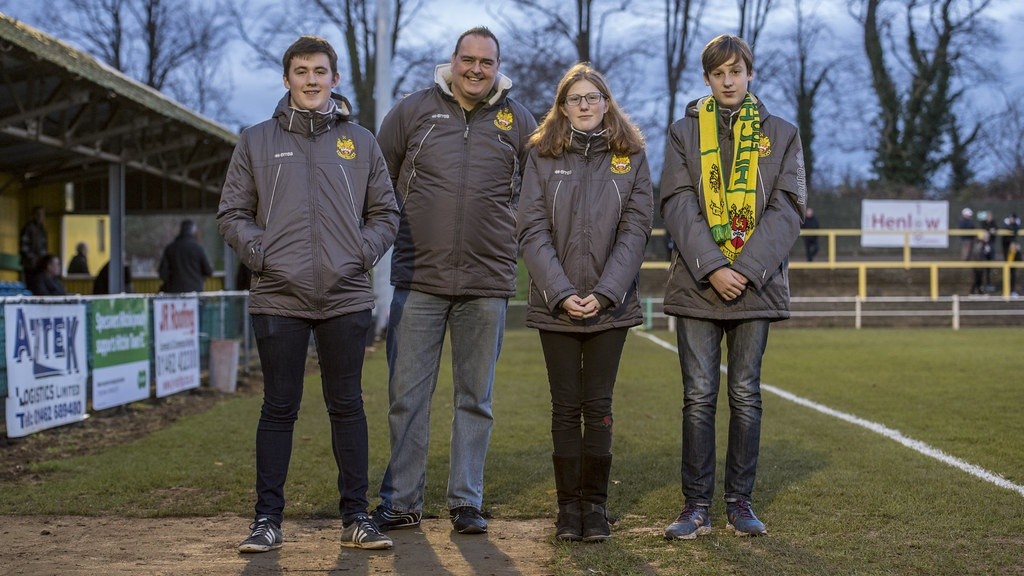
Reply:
[726,499,767,536]
[450,506,488,533]
[368,504,422,532]
[238,513,283,552]
[340,516,393,549]
[664,501,712,539]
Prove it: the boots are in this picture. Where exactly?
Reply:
[552,452,582,541]
[581,448,612,542]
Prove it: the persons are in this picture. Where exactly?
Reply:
[68,244,89,273]
[973,244,991,294]
[982,211,997,259]
[216,36,401,552]
[29,254,65,295]
[93,261,131,295]
[1006,242,1022,298]
[369,27,538,533]
[659,35,808,540]
[959,208,976,260]
[800,207,820,262]
[158,219,211,292]
[515,64,654,541]
[1001,214,1022,260]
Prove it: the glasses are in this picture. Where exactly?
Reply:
[562,91,609,106]
